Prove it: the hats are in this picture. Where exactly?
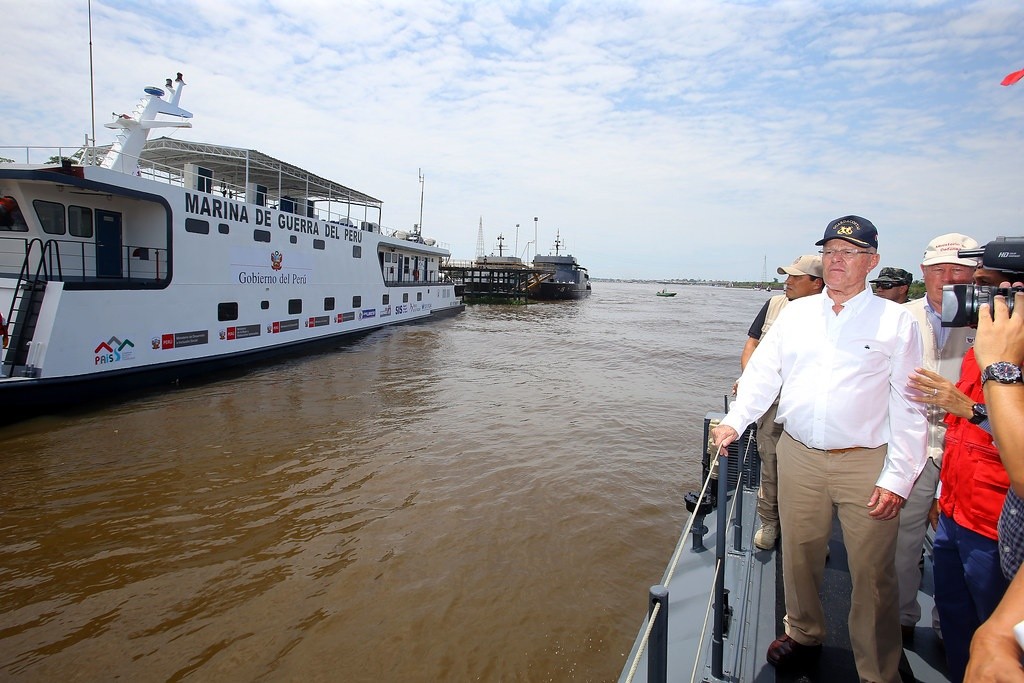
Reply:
[869,267,913,284]
[777,254,824,278]
[816,215,879,249]
[922,233,980,267]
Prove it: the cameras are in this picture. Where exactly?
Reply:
[941,283,1024,329]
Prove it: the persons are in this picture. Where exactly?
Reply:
[711,215,1024,683]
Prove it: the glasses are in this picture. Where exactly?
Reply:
[875,283,904,290]
[818,248,874,258]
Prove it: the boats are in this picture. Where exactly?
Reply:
[655,291,677,297]
[464,232,531,301]
[0,71,467,391]
[442,263,471,297]
[527,229,593,300]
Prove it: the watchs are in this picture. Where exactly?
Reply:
[981,362,1023,388]
[968,402,988,425]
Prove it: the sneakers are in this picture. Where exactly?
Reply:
[766,634,822,667]
[754,522,778,549]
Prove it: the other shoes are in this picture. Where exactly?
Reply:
[900,625,915,637]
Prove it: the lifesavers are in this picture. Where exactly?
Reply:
[415,269,420,280]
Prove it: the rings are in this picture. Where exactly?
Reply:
[933,388,937,396]
